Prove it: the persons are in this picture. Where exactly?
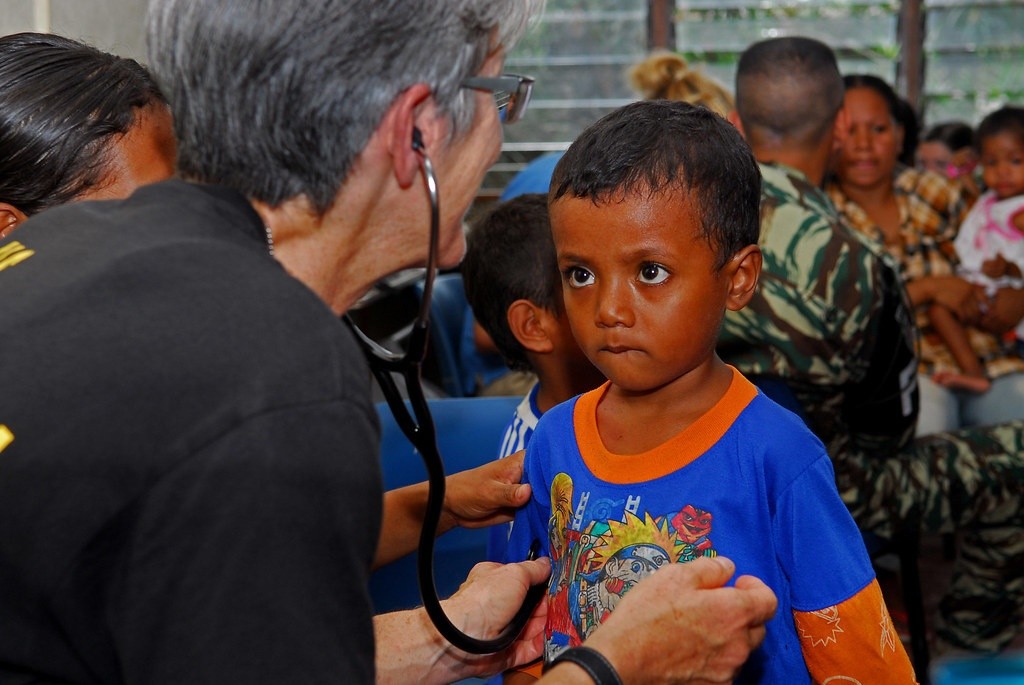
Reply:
[0,0,1024,684]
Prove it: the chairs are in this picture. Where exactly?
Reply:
[372,396,530,685]
[418,273,469,397]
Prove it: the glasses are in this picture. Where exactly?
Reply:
[460,74,536,124]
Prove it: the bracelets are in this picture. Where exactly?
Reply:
[540,644,621,684]
